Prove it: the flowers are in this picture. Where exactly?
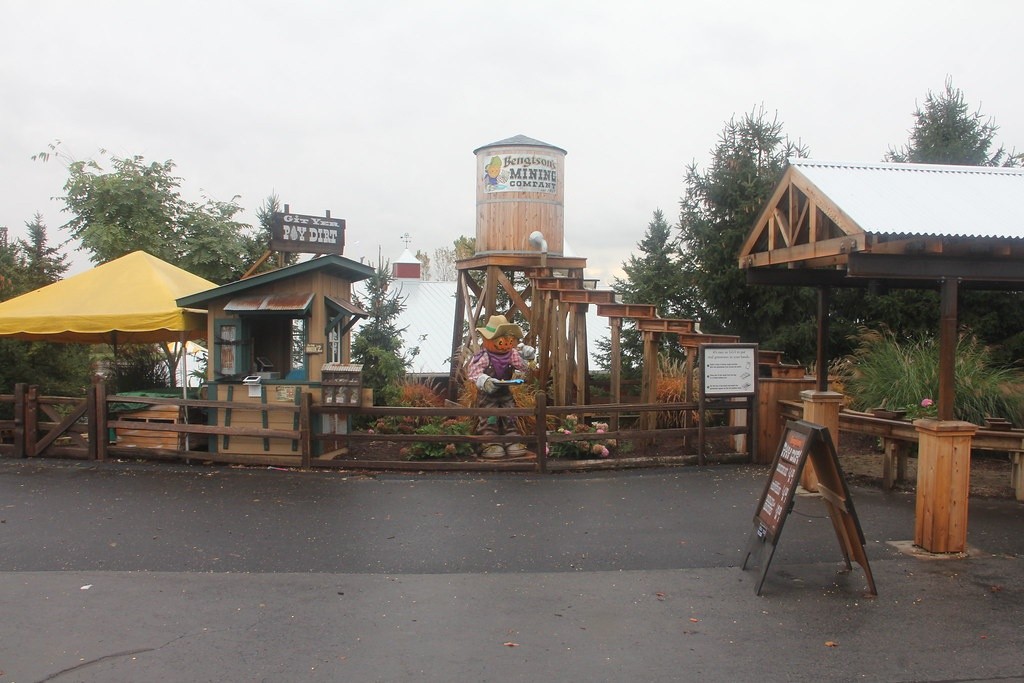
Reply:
[549,414,616,458]
[906,399,935,419]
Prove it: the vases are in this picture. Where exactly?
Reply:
[567,448,582,460]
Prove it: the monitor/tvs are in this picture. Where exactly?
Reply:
[257,357,274,367]
[242,376,262,382]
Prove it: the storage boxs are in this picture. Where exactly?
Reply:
[256,371,281,380]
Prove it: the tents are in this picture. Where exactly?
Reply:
[0,250,220,463]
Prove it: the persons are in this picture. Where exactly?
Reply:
[467,315,536,458]
